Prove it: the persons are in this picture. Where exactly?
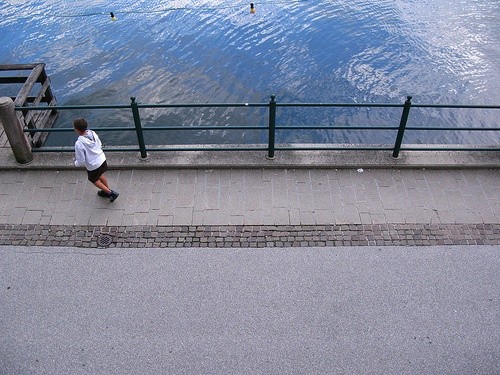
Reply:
[72,118,119,202]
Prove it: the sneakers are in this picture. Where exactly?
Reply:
[98,190,110,197]
[110,190,118,202]
